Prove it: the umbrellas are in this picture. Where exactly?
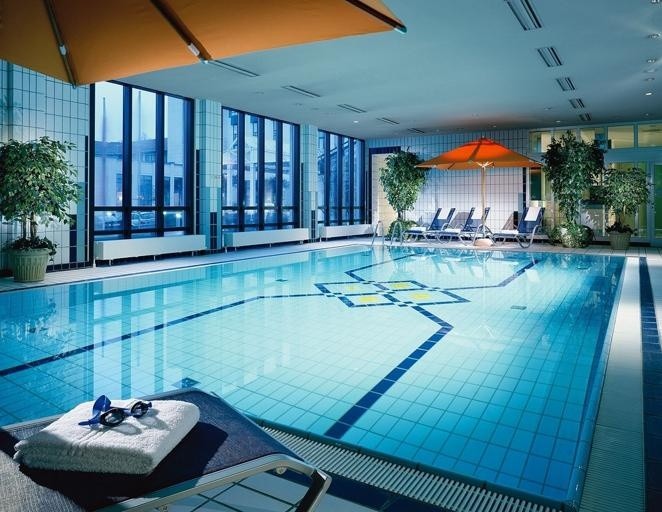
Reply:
[0,0,405,87]
[414,135,546,237]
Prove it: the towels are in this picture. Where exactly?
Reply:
[13,397,202,475]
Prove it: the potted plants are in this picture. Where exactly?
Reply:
[544,131,650,250]
[380,150,428,240]
[1,135,77,282]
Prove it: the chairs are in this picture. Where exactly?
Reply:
[404,205,547,249]
[0,385,331,512]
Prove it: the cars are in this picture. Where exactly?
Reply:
[105,203,324,230]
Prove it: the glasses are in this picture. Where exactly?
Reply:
[78,395,152,427]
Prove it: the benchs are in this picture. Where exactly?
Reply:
[94,224,375,262]
